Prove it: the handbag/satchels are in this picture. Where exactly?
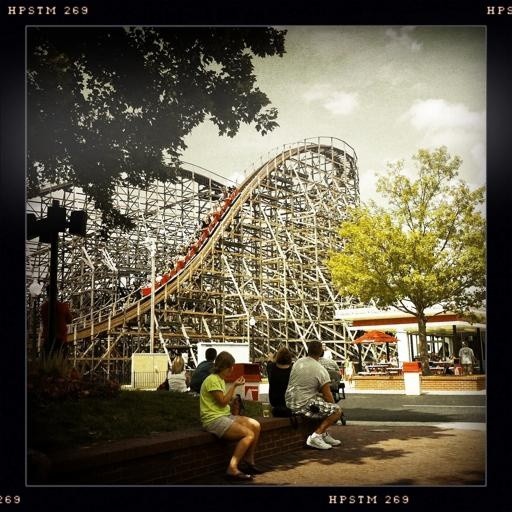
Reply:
[271,407,292,418]
[156,379,169,391]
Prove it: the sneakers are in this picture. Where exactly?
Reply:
[306,435,332,450]
[322,432,341,447]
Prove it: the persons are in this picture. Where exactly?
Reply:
[284,341,343,450]
[344,355,356,381]
[199,351,264,481]
[167,355,191,393]
[365,350,398,368]
[431,352,438,361]
[438,342,450,371]
[40,284,73,367]
[266,347,292,417]
[190,348,217,393]
[131,185,236,303]
[459,340,476,375]
[321,342,333,360]
[318,349,343,403]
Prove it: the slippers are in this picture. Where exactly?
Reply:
[242,463,262,473]
[223,473,252,481]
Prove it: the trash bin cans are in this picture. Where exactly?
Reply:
[225,363,261,401]
[402,361,422,396]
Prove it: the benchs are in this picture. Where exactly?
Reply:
[358,360,480,376]
